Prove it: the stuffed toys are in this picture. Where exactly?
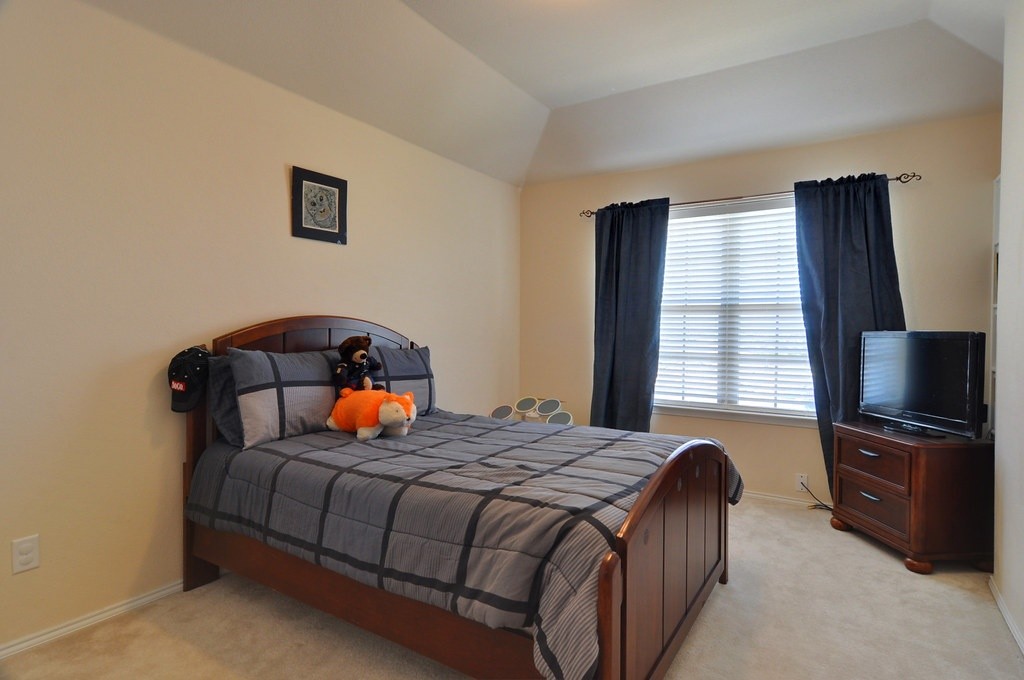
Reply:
[333,336,385,391]
[326,388,417,442]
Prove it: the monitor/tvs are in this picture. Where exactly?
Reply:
[858,330,987,440]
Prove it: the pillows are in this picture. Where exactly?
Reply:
[227,346,337,450]
[206,353,245,448]
[322,345,438,416]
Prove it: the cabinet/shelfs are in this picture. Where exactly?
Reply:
[830,417,994,574]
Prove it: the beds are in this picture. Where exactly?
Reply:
[171,314,744,680]
